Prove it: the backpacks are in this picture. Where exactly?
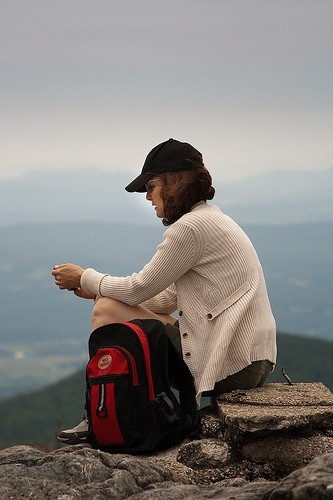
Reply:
[84,317,202,454]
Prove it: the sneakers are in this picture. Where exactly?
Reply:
[55,416,89,446]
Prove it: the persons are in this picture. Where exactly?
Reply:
[51,139,276,443]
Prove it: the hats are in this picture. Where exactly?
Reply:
[125,138,203,194]
